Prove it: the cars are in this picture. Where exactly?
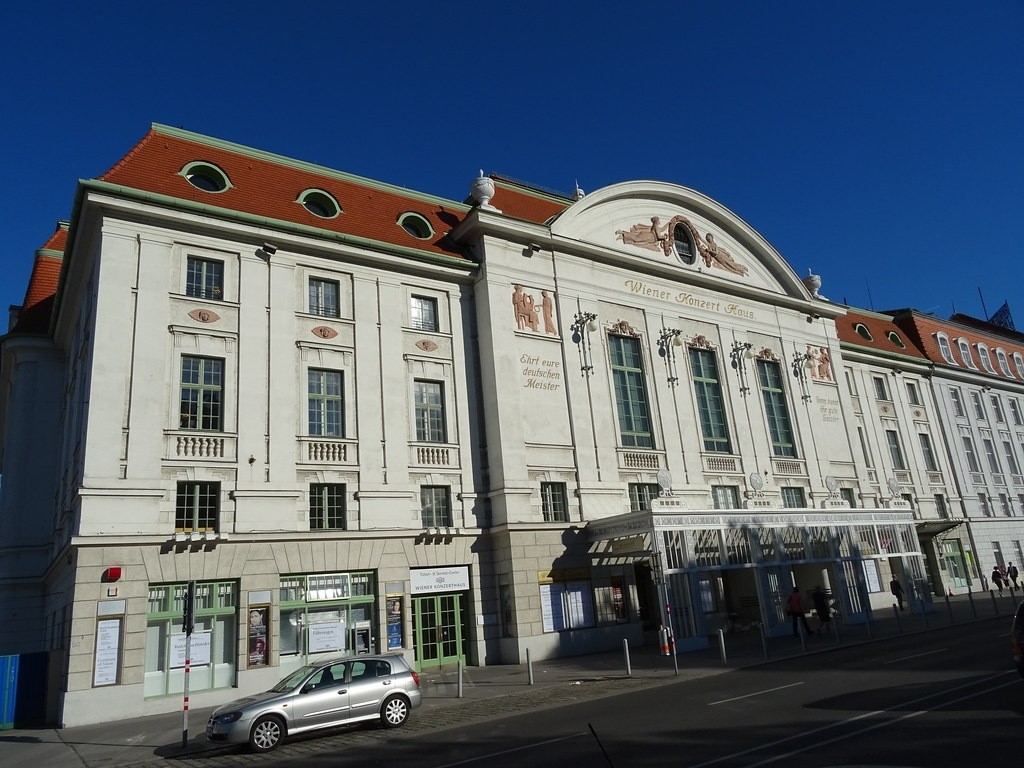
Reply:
[1013,600,1024,674]
[205,651,423,753]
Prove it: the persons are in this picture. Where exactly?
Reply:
[764,348,771,359]
[199,312,210,321]
[250,610,263,626]
[320,328,330,337]
[1001,562,1020,589]
[512,285,557,335]
[620,321,629,333]
[788,587,815,637]
[812,586,831,632]
[423,342,431,350]
[890,574,904,611]
[250,641,265,654]
[391,601,400,614]
[697,232,749,277]
[615,216,672,245]
[698,335,705,346]
[807,346,831,380]
[992,566,1004,598]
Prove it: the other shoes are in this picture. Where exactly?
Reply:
[794,633,800,637]
[816,629,822,637]
[807,630,815,635]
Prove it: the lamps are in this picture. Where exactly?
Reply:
[456,527,465,534]
[175,532,186,542]
[792,350,814,403]
[219,533,228,540]
[660,327,683,386]
[731,341,755,395]
[575,312,598,377]
[446,527,457,535]
[438,527,447,535]
[205,531,216,540]
[426,527,437,535]
[190,531,201,541]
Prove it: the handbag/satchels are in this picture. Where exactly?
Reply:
[993,571,996,581]
